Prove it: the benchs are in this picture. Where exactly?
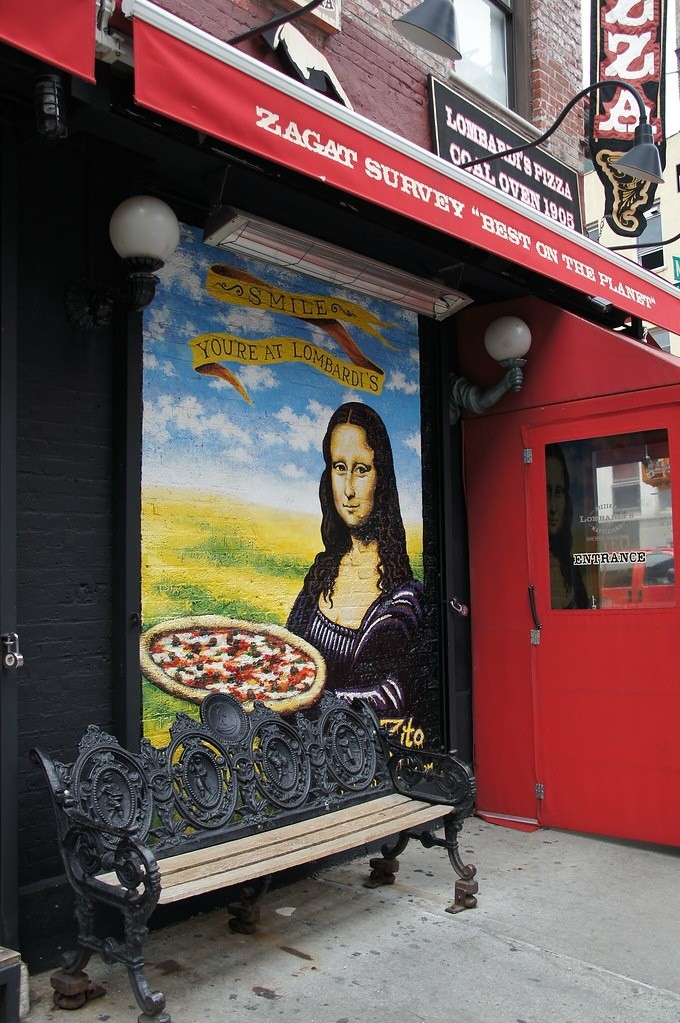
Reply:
[29,692,480,1023]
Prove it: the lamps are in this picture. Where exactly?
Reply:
[35,72,67,141]
[458,80,665,188]
[447,315,531,422]
[225,0,462,62]
[65,196,179,335]
[201,161,473,321]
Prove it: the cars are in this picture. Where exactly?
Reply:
[600,546,675,609]
